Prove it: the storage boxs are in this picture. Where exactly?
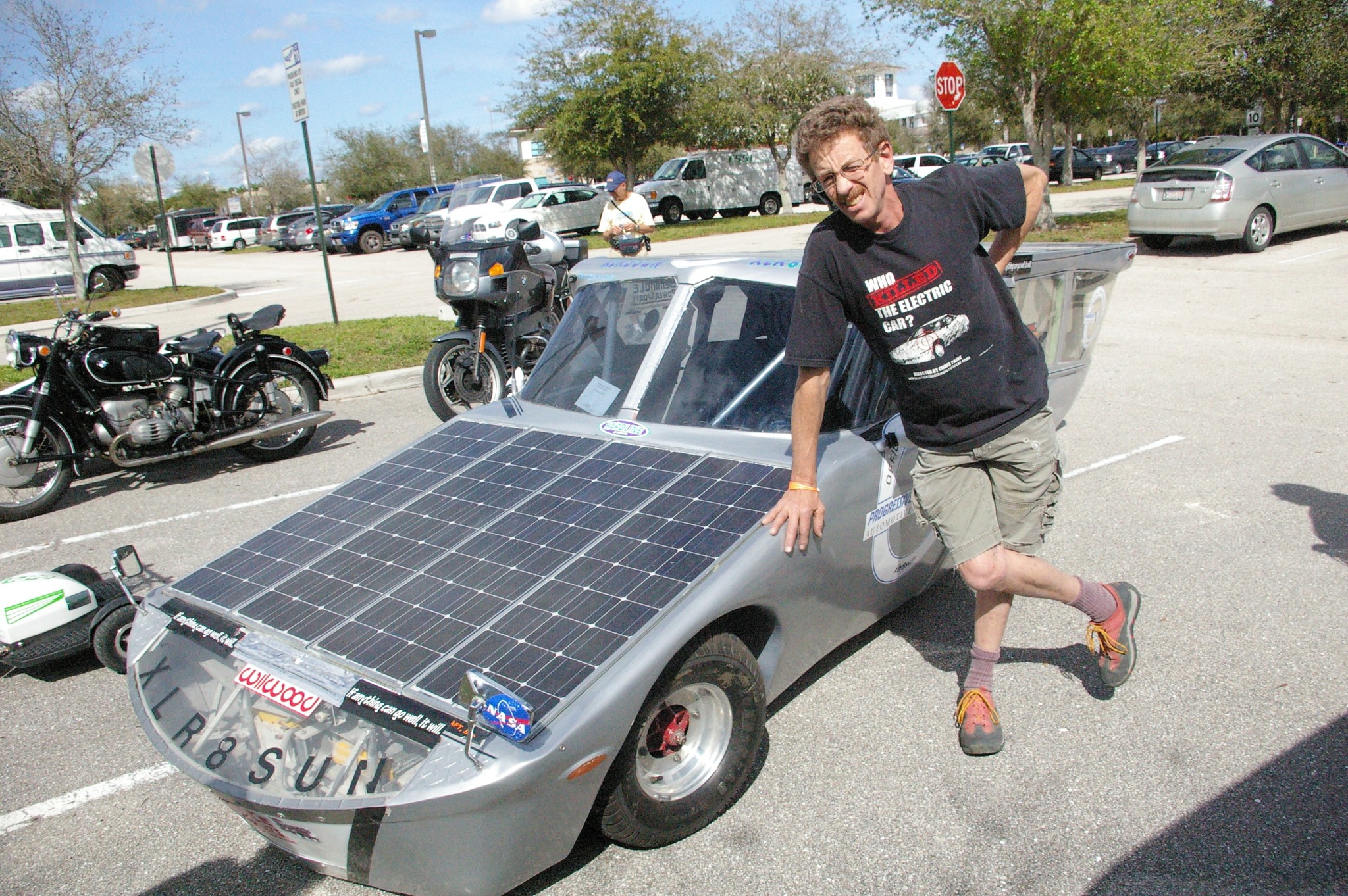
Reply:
[564,239,588,263]
[94,323,160,351]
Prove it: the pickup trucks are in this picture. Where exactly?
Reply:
[410,220,594,425]
[422,177,568,248]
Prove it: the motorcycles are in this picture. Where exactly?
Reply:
[0,264,337,522]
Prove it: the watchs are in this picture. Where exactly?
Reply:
[634,224,640,231]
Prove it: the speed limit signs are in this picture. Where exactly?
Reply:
[1247,105,1264,127]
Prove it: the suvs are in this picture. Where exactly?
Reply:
[259,209,337,252]
[208,216,271,252]
[390,187,484,251]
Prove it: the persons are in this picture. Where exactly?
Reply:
[598,171,657,258]
[760,98,1140,755]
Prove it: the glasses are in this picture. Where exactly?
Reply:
[608,183,621,193]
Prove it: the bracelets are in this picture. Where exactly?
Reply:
[788,481,820,492]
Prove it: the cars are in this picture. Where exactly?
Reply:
[139,230,161,251]
[1127,133,1348,254]
[892,134,1231,178]
[1023,146,1103,184]
[592,182,609,191]
[538,183,591,189]
[471,185,613,243]
[114,239,1140,892]
[284,203,373,254]
[114,232,143,248]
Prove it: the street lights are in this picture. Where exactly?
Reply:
[415,28,438,185]
[236,111,256,210]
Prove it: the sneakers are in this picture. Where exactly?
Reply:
[1086,581,1142,687]
[954,685,1005,755]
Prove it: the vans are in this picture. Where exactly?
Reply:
[0,209,141,295]
[187,216,230,251]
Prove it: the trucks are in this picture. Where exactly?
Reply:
[632,145,804,226]
[154,207,217,252]
[330,177,505,256]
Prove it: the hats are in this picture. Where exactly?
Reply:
[606,170,626,191]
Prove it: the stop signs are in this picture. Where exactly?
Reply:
[936,61,964,111]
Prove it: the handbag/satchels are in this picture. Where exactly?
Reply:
[645,236,651,251]
[611,236,645,254]
